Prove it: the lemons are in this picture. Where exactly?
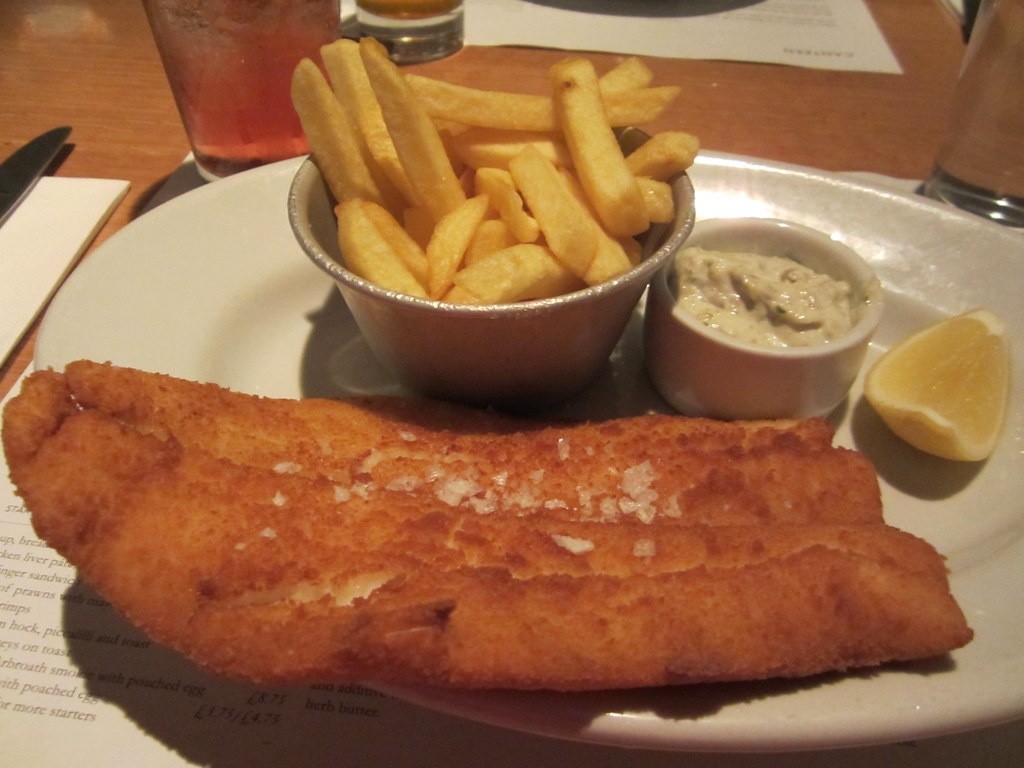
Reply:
[866,308,1013,462]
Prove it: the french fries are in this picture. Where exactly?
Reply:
[289,33,700,304]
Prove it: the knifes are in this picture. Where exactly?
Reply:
[0,125,72,224]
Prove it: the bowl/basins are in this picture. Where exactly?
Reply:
[642,218,887,427]
[289,124,696,409]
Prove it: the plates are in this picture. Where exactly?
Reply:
[36,148,1024,754]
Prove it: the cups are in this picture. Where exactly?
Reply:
[354,0,465,66]
[142,0,343,185]
[923,0,1024,229]
